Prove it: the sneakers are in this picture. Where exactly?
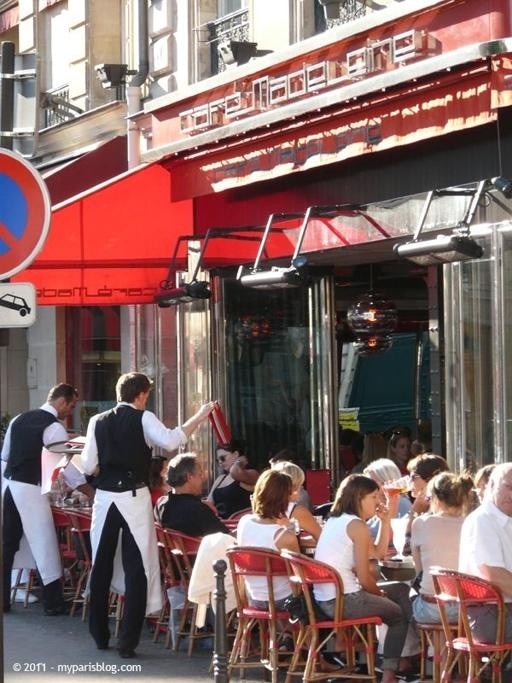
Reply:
[117,648,137,658]
[45,602,80,617]
[97,629,110,649]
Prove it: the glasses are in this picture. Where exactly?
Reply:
[215,455,227,462]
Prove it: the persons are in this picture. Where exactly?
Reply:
[10,568,38,603]
[80,372,218,658]
[1,383,80,615]
[71,527,92,596]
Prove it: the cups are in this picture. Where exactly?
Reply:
[384,476,410,500]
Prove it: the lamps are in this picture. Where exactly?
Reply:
[219,39,274,65]
[154,223,283,311]
[94,63,138,89]
[393,177,497,264]
[236,200,365,290]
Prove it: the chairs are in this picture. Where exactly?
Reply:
[44,468,511,683]
